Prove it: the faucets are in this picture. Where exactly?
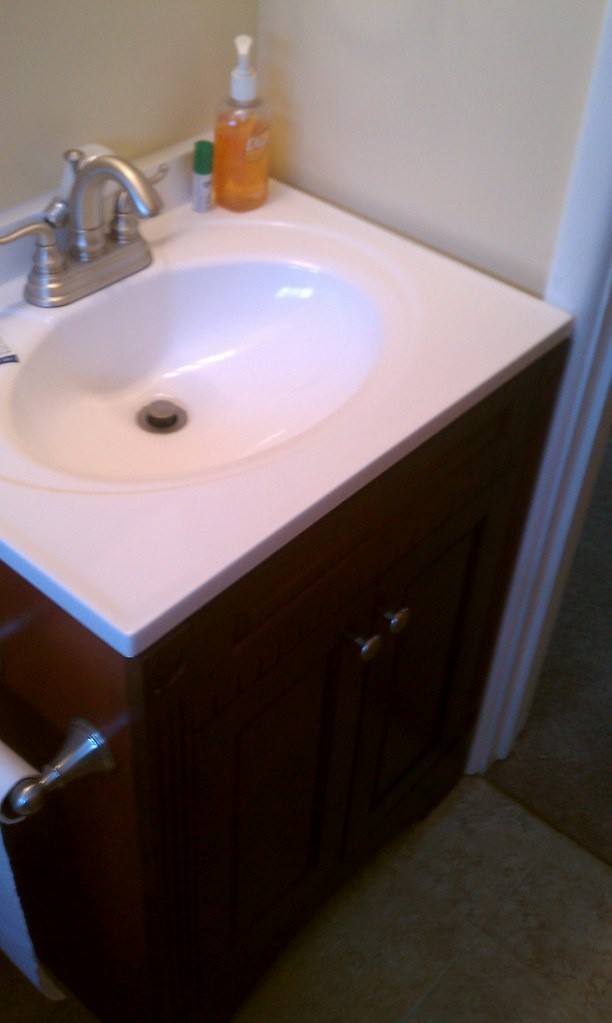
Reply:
[64,155,162,263]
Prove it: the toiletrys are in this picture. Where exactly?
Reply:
[191,141,214,213]
[214,35,271,213]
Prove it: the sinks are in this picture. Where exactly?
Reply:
[0,218,414,492]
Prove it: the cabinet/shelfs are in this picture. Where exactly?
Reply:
[0,342,574,1023]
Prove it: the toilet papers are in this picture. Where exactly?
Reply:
[0,739,67,1000]
